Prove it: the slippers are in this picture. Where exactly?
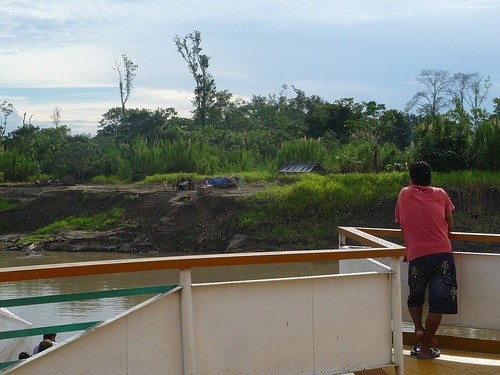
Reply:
[411,345,422,356]
[416,347,440,359]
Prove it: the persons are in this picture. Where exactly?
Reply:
[32,333,57,355]
[38,339,54,352]
[18,351,32,359]
[395,161,459,360]
